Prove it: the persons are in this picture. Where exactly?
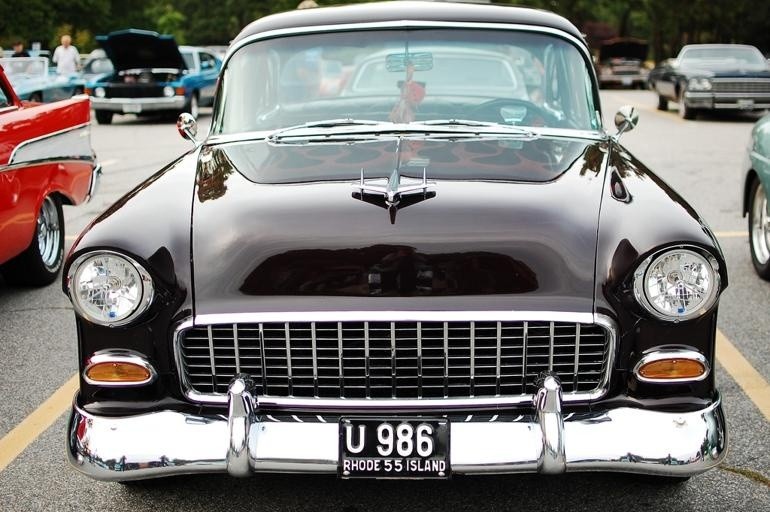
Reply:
[11,42,32,73]
[51,34,83,75]
